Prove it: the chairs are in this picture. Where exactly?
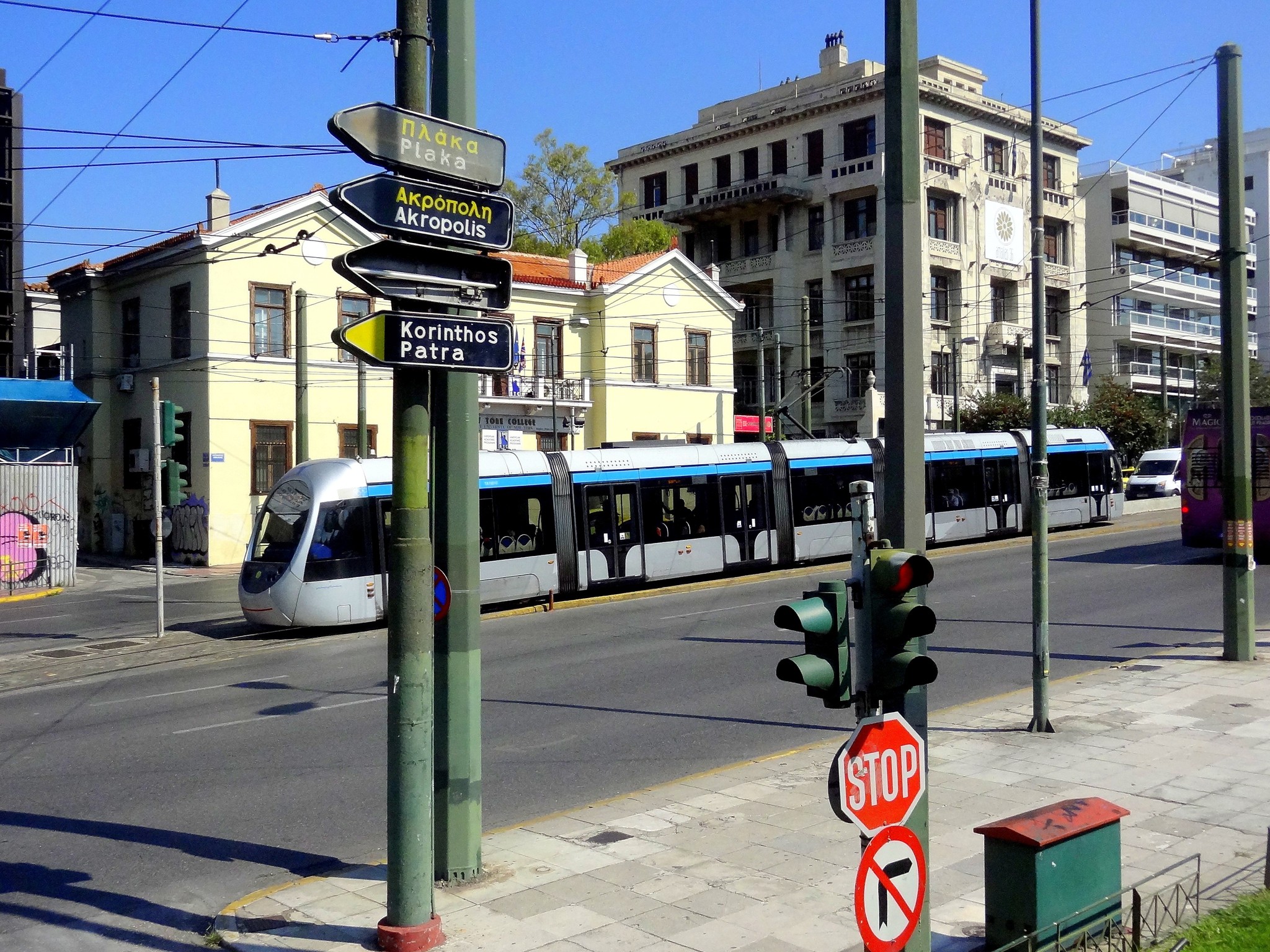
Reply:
[660,523,669,537]
[1068,483,1076,494]
[498,536,515,554]
[816,504,827,520]
[803,506,815,521]
[685,521,691,534]
[515,534,533,552]
[955,495,963,506]
[835,504,843,518]
[1061,484,1068,495]
[1047,485,1053,497]
[479,538,493,557]
[941,496,949,507]
[1053,485,1060,496]
[845,503,852,517]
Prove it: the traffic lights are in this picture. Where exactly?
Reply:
[163,400,185,449]
[773,590,849,709]
[869,547,939,705]
[162,459,187,508]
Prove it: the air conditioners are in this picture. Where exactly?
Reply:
[116,374,134,391]
[129,448,149,473]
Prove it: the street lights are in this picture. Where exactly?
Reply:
[1177,349,1207,448]
[549,317,591,448]
[939,336,980,429]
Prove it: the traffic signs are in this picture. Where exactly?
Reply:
[332,99,508,191]
[338,312,517,378]
[334,173,514,252]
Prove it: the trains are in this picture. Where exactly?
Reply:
[238,364,1126,629]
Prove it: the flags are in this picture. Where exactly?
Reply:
[1081,349,1093,387]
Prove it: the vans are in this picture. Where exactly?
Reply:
[1125,448,1182,500]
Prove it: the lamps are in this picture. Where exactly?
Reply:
[483,404,491,409]
[569,318,590,329]
[534,407,543,412]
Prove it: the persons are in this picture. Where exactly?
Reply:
[681,526,690,535]
[943,488,964,506]
[697,524,705,533]
[661,499,695,524]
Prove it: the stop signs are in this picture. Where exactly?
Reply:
[837,712,925,839]
